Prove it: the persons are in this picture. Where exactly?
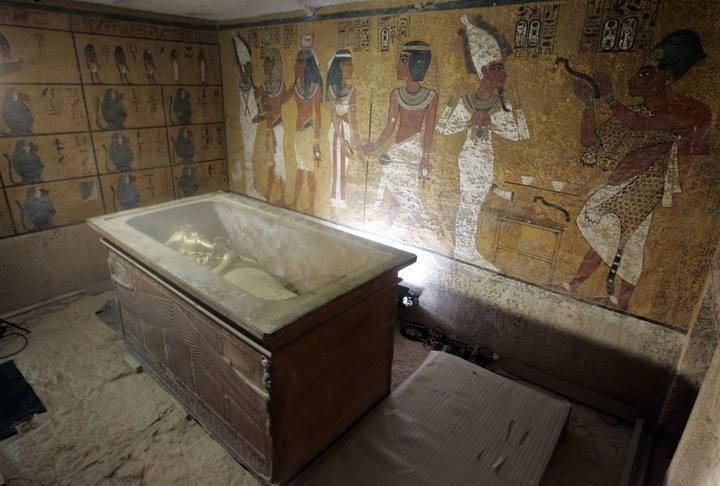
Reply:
[238,47,286,209]
[115,45,133,86]
[324,50,359,223]
[198,50,209,85]
[541,62,712,313]
[260,48,323,217]
[143,48,159,86]
[356,40,438,249]
[434,61,531,275]
[171,49,182,85]
[85,44,103,85]
[167,228,299,300]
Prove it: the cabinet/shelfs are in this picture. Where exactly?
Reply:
[86,190,418,486]
